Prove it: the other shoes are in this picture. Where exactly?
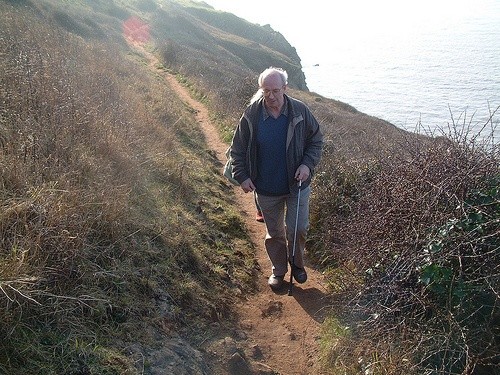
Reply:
[291,258,307,284]
[268,274,285,289]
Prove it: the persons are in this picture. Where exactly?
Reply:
[228,67,323,290]
[248,88,264,223]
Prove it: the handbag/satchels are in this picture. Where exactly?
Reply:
[224,114,254,185]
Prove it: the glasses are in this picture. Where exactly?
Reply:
[263,84,285,97]
